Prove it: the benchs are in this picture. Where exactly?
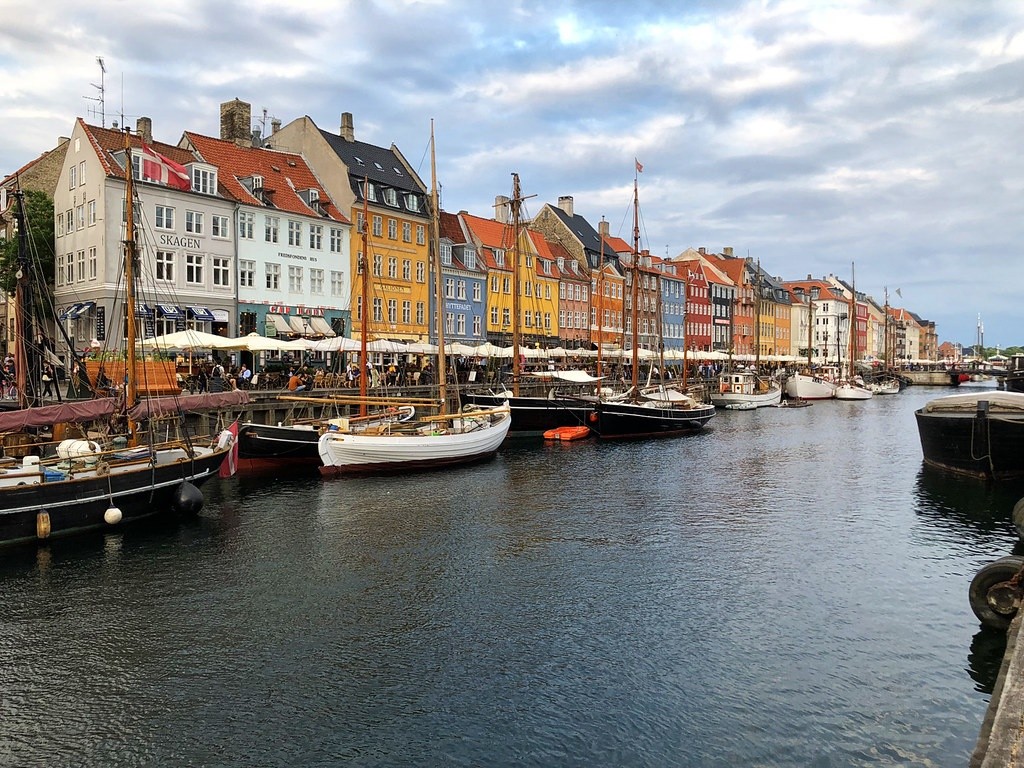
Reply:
[86,362,180,398]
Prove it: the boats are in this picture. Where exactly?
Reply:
[914,388,1024,484]
[1004,352,1024,392]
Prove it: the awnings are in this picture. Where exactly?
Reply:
[155,305,185,319]
[187,305,216,321]
[124,303,154,319]
[289,316,316,338]
[264,314,292,337]
[311,317,337,338]
[59,301,95,321]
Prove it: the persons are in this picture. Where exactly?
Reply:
[195,358,434,397]
[42,360,53,397]
[95,367,116,397]
[0,352,15,399]
[124,368,141,399]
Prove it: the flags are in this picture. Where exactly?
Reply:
[636,160,643,173]
[895,288,902,299]
[141,140,190,191]
[213,419,238,478]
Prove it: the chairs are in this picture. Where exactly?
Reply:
[394,372,421,386]
[314,371,361,389]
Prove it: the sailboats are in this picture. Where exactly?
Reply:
[0,120,962,553]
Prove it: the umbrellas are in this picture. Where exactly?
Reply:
[233,332,306,374]
[407,342,734,363]
[135,328,249,373]
[286,338,327,366]
[362,340,424,373]
[314,336,368,374]
[731,353,833,363]
